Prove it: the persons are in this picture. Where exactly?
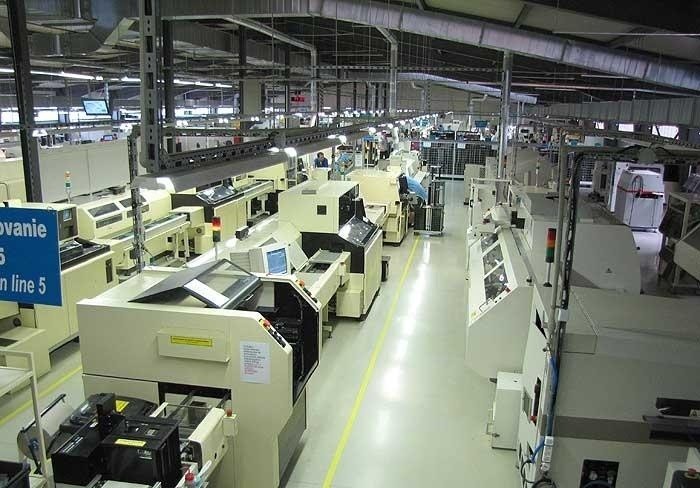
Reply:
[405,129,408,136]
[314,152,328,167]
[380,132,389,159]
[411,129,414,137]
[402,173,427,229]
[438,125,444,131]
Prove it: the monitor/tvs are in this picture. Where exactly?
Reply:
[230,242,291,275]
[82,99,109,115]
